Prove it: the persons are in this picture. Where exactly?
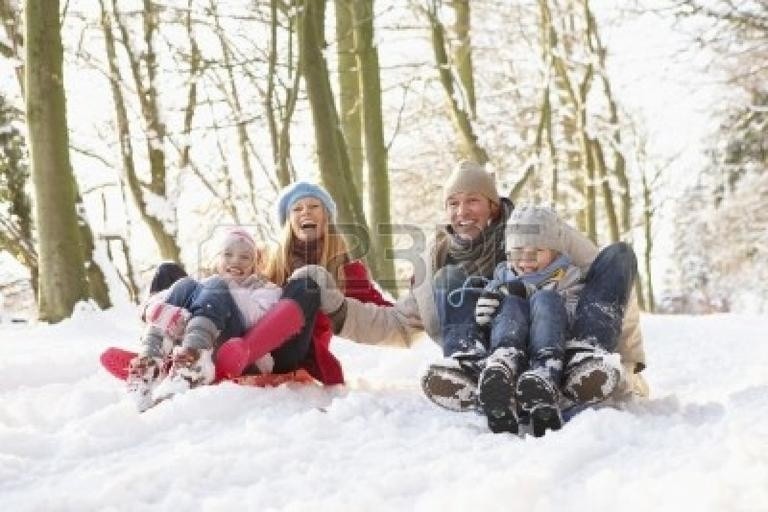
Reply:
[127,230,287,414]
[99,179,415,391]
[287,156,649,420]
[473,204,586,439]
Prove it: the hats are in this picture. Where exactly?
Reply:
[274,179,336,226]
[442,157,501,207]
[499,199,566,256]
[217,228,258,260]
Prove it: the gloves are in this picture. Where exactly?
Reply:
[287,263,344,317]
[473,288,503,328]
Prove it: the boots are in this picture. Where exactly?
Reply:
[96,343,166,379]
[512,347,567,438]
[211,295,307,376]
[560,348,625,419]
[126,323,175,413]
[477,350,521,434]
[165,314,220,393]
[419,350,484,417]
